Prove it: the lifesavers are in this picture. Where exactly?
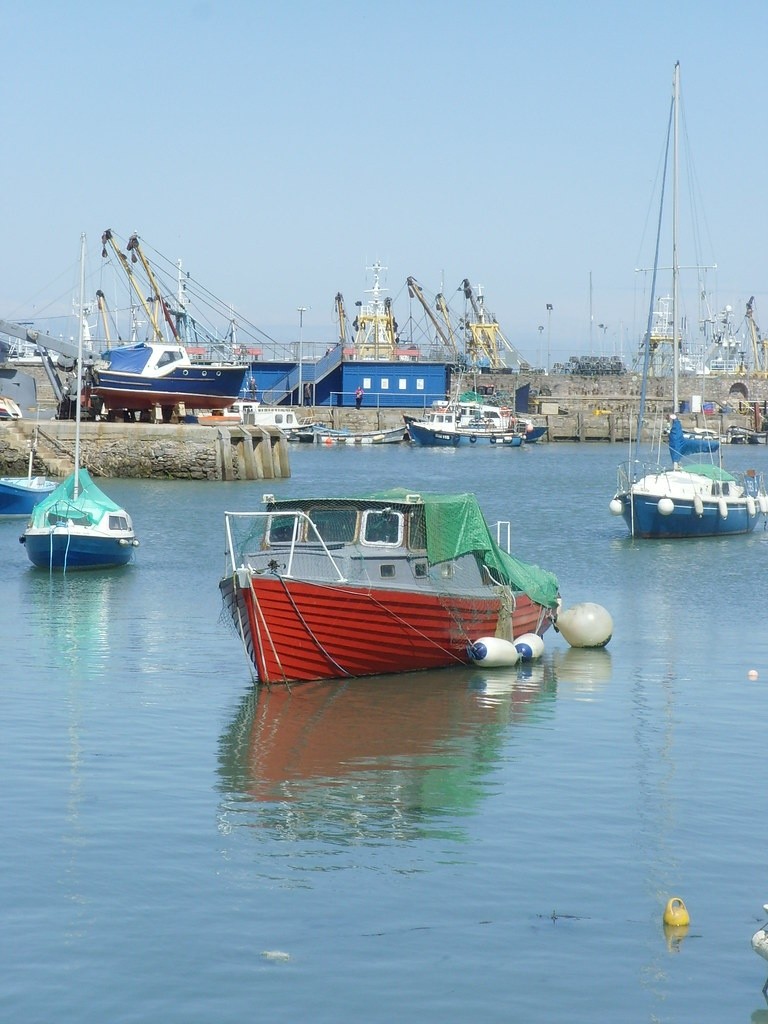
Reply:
[490,436,496,444]
[470,435,477,443]
[452,433,462,445]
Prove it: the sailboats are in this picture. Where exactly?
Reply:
[0,400,61,520]
[612,53,768,540]
[19,230,142,574]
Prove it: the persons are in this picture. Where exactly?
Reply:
[250,378,258,400]
[355,386,364,410]
[303,383,311,407]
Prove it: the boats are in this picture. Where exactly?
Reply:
[180,355,551,449]
[215,487,563,689]
[238,643,554,816]
[68,228,303,421]
[197,415,243,427]
